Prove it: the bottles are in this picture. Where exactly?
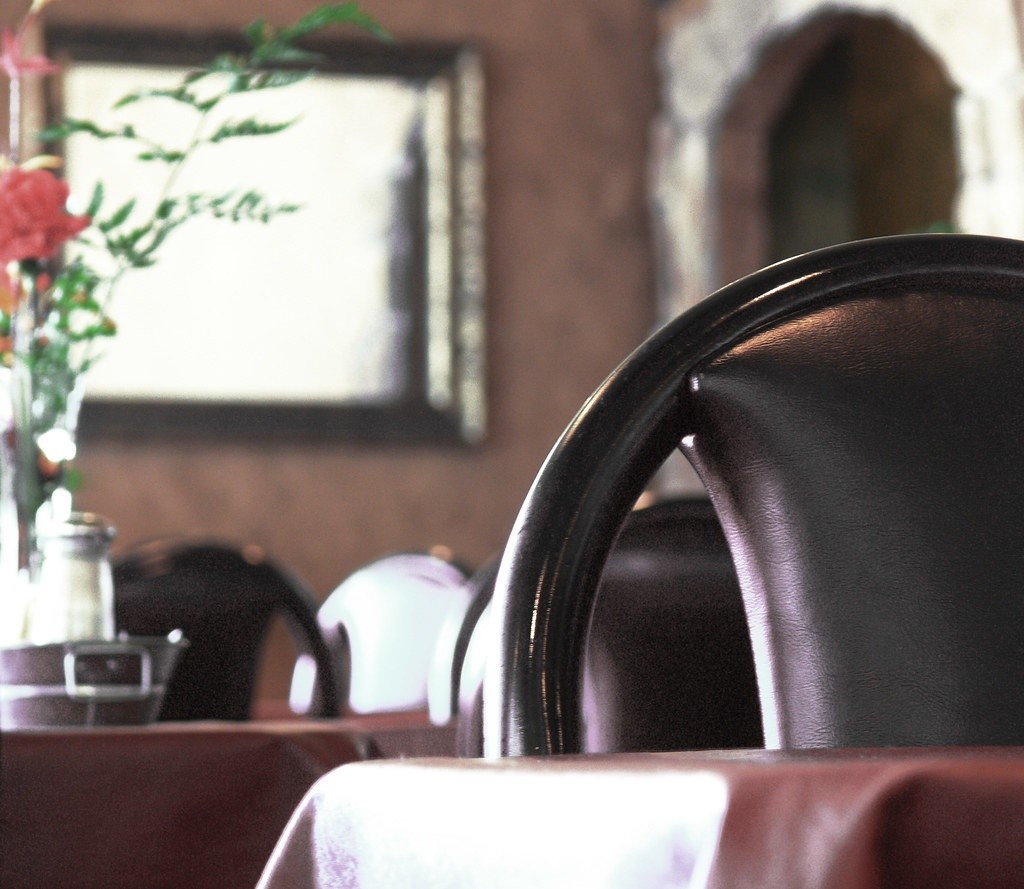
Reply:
[31,512,119,646]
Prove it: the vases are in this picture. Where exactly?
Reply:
[0,372,91,643]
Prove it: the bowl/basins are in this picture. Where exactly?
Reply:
[0,634,193,727]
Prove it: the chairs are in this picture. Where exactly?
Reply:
[112,534,343,719]
[423,487,758,750]
[487,231,1024,746]
[284,552,476,714]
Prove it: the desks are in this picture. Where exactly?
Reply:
[260,741,1024,889]
[0,717,466,889]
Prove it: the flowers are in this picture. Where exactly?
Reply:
[0,0,402,595]
